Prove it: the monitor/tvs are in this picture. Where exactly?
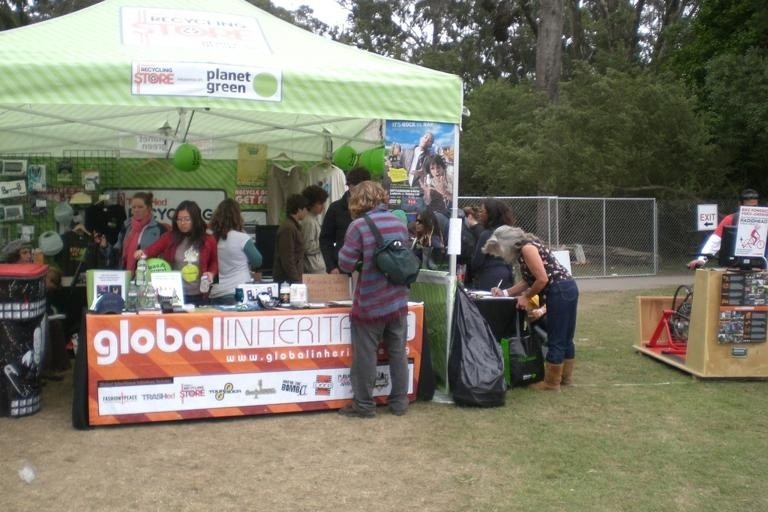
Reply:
[252,224,282,275]
[719,225,764,269]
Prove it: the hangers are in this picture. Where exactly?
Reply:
[317,144,336,168]
[73,213,94,241]
[93,189,117,208]
[133,158,172,176]
[268,144,300,167]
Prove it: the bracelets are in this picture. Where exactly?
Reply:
[537,309,545,315]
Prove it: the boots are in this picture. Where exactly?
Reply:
[563,358,575,385]
[529,362,562,391]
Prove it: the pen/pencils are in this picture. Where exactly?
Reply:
[494,279,503,297]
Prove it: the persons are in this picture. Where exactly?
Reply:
[526,292,547,357]
[320,167,371,274]
[92,186,328,300]
[481,220,577,392]
[5,238,67,384]
[401,132,435,187]
[420,159,453,206]
[338,181,411,417]
[686,189,761,270]
[412,196,522,293]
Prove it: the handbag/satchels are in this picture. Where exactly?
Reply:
[373,239,422,285]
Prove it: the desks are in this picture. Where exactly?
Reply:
[85,301,425,428]
[467,290,522,342]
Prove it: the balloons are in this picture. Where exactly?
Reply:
[335,147,357,170]
[173,145,201,171]
[358,147,374,175]
[371,147,385,174]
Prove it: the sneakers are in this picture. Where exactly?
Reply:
[384,402,408,415]
[338,404,375,418]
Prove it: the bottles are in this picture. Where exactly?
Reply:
[199,273,211,294]
[458,264,465,287]
[279,280,291,307]
[128,253,156,311]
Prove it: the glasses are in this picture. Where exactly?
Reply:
[177,216,192,224]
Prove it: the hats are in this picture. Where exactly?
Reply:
[38,231,63,256]
[70,192,93,205]
[741,188,759,198]
[91,293,124,315]
[54,202,74,225]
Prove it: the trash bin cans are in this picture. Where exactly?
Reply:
[551,249,572,277]
